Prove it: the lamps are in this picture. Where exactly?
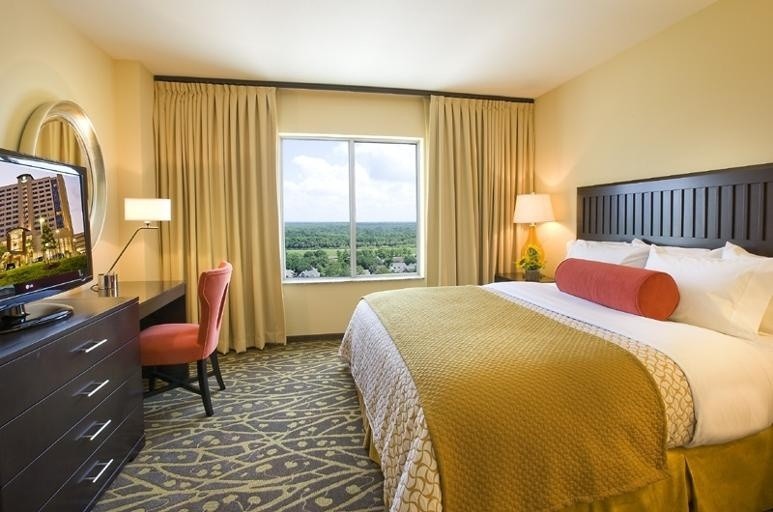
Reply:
[91,198,171,290]
[512,192,556,273]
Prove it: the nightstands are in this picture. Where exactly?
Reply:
[495,273,555,282]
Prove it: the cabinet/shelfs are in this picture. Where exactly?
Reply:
[1,296,146,511]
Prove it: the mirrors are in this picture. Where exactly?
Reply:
[18,100,108,251]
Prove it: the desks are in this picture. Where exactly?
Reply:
[67,281,189,381]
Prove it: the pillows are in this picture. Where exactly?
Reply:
[632,238,711,252]
[726,241,773,334]
[567,240,657,268]
[555,258,680,320]
[643,244,773,341]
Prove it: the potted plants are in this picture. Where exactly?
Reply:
[513,246,546,281]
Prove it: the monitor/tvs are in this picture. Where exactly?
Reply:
[0,148,93,332]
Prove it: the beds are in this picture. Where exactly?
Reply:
[339,163,773,510]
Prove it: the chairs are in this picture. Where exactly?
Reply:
[140,261,232,416]
